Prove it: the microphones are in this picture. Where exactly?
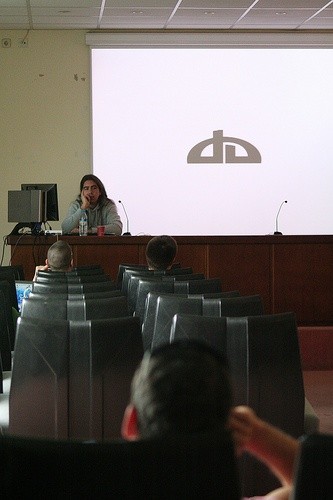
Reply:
[119,200,130,236]
[274,200,288,234]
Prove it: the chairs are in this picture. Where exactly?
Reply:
[0,265,332,500]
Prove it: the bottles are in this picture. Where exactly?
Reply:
[78,209,88,237]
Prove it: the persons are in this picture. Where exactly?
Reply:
[33,240,75,283]
[61,175,123,236]
[144,234,178,271]
[119,338,298,500]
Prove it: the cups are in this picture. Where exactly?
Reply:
[96,226,106,236]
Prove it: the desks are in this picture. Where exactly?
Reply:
[6,233,332,327]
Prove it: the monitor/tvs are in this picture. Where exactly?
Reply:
[20,184,59,222]
